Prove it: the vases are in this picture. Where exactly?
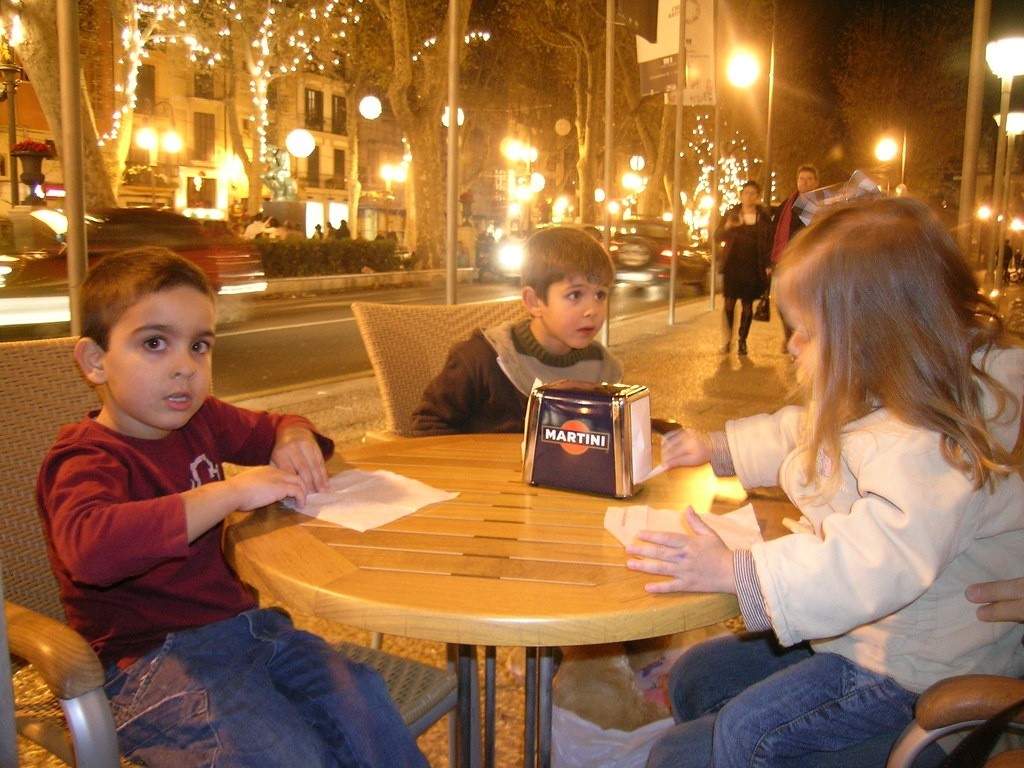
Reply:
[10,151,46,205]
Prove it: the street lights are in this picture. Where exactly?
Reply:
[978,37,1024,304]
[523,144,537,234]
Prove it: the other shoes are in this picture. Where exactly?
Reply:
[738,339,748,354]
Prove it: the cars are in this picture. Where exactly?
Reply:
[487,214,710,293]
[61,205,209,261]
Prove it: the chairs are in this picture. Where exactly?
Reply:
[886,673,1024,768]
[0,335,459,768]
[351,298,536,768]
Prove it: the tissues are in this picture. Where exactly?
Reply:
[519,375,670,500]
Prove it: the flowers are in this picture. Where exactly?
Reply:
[10,138,55,161]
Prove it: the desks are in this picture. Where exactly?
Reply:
[222,430,800,768]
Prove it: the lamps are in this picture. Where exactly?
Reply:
[194,171,206,191]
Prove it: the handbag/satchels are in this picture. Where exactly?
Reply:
[754,274,771,322]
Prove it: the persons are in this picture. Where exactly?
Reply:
[35,247,430,768]
[766,164,819,355]
[995,239,1022,283]
[457,230,496,270]
[624,195,1024,768]
[376,227,398,242]
[244,213,350,242]
[411,226,615,437]
[714,180,772,356]
